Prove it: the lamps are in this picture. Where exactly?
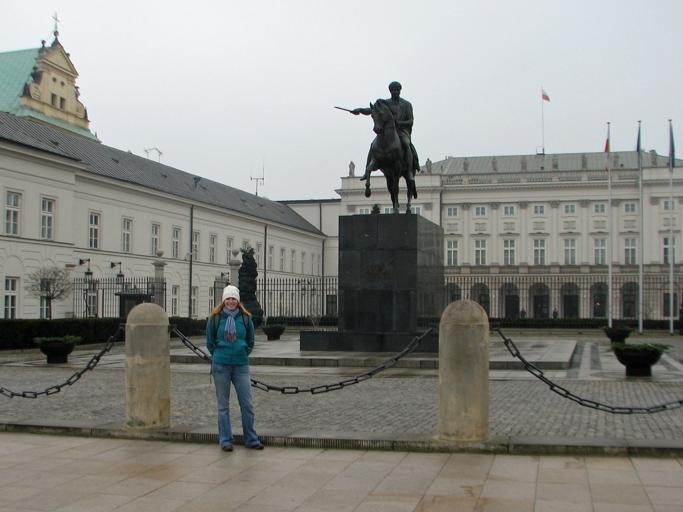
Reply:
[110,261,124,278]
[79,258,94,275]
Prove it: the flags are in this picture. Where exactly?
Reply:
[542,88,550,102]
[667,124,675,168]
[604,137,610,161]
[637,127,640,153]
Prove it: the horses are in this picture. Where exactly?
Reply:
[360,97,419,214]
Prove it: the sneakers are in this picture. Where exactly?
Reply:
[250,444,263,450]
[223,445,233,452]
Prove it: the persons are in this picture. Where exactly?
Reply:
[651,150,657,166]
[582,154,587,168]
[426,158,432,173]
[613,152,619,168]
[552,154,559,170]
[350,81,420,199]
[463,157,469,172]
[205,285,265,451]
[492,157,498,172]
[520,156,528,171]
[349,161,355,177]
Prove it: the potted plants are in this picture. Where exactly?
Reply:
[602,340,673,378]
[34,335,84,364]
[261,323,286,340]
[601,326,633,343]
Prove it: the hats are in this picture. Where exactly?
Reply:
[221,285,240,304]
